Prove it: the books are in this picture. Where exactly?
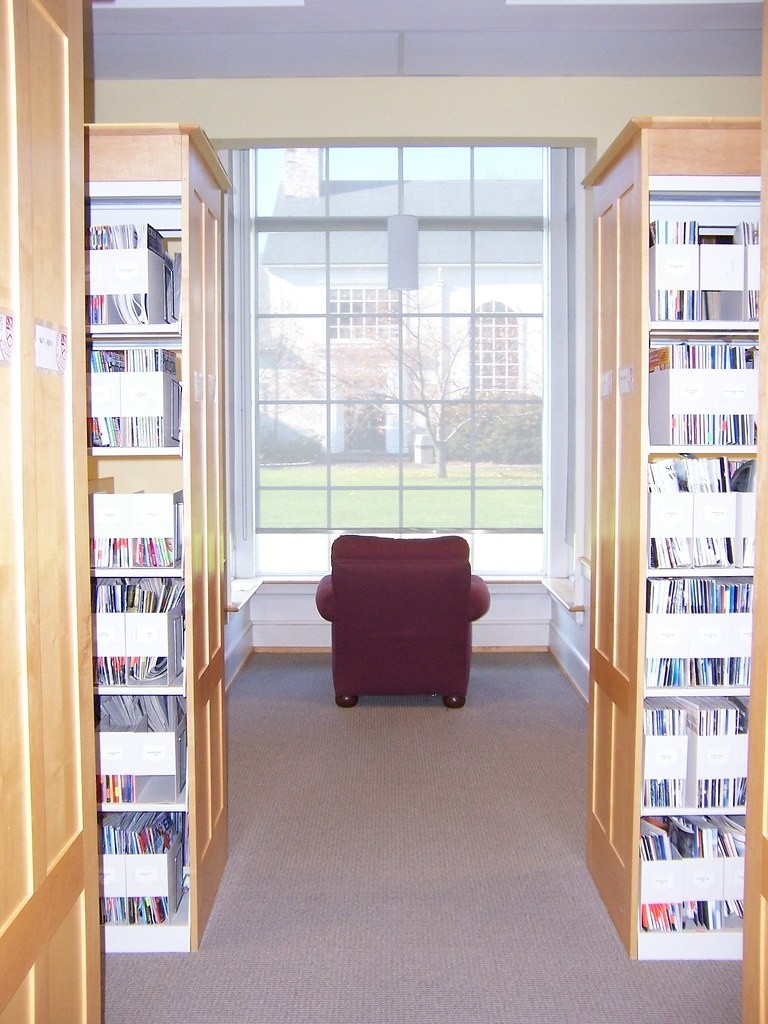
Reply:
[90,501,184,568]
[645,577,753,689]
[99,812,189,926]
[641,696,750,808]
[648,459,757,570]
[648,343,760,446]
[90,577,185,686]
[94,695,187,804]
[649,219,759,321]
[640,816,746,933]
[85,222,181,325]
[86,349,179,447]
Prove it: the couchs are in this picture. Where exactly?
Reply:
[314,533,494,709]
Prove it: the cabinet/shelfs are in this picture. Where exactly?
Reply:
[0,0,230,1024]
[578,115,768,1024]
